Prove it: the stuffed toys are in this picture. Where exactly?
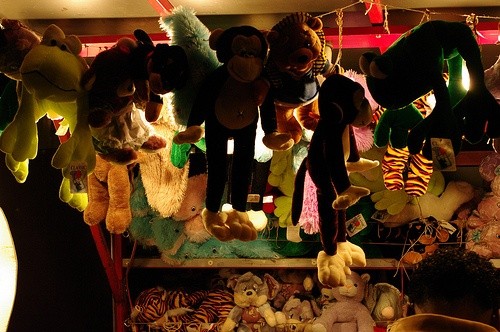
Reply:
[83,38,163,164]
[0,19,96,211]
[266,11,344,145]
[358,20,500,157]
[134,28,196,124]
[174,25,294,242]
[291,74,380,287]
[84,123,500,332]
